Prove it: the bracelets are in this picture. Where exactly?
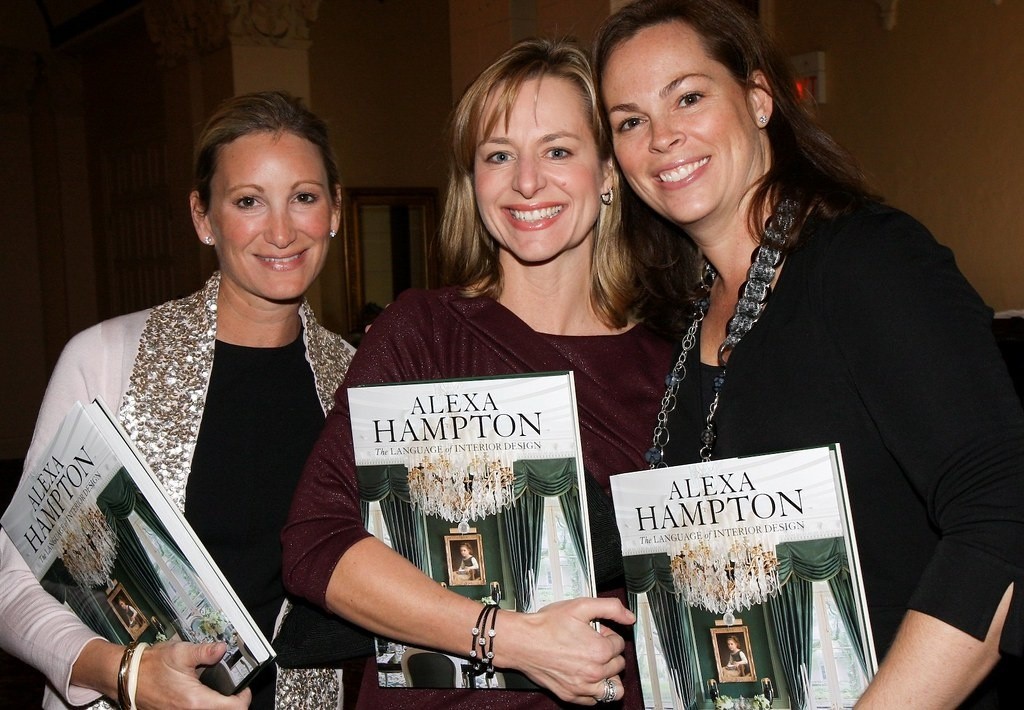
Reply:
[470,603,501,674]
[117,640,137,709]
[127,642,152,710]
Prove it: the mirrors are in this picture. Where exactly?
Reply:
[336,185,445,331]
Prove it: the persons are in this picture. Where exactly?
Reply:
[282,35,704,710]
[592,0,1023,710]
[721,635,750,676]
[0,84,365,710]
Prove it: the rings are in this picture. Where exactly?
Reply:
[598,678,616,703]
